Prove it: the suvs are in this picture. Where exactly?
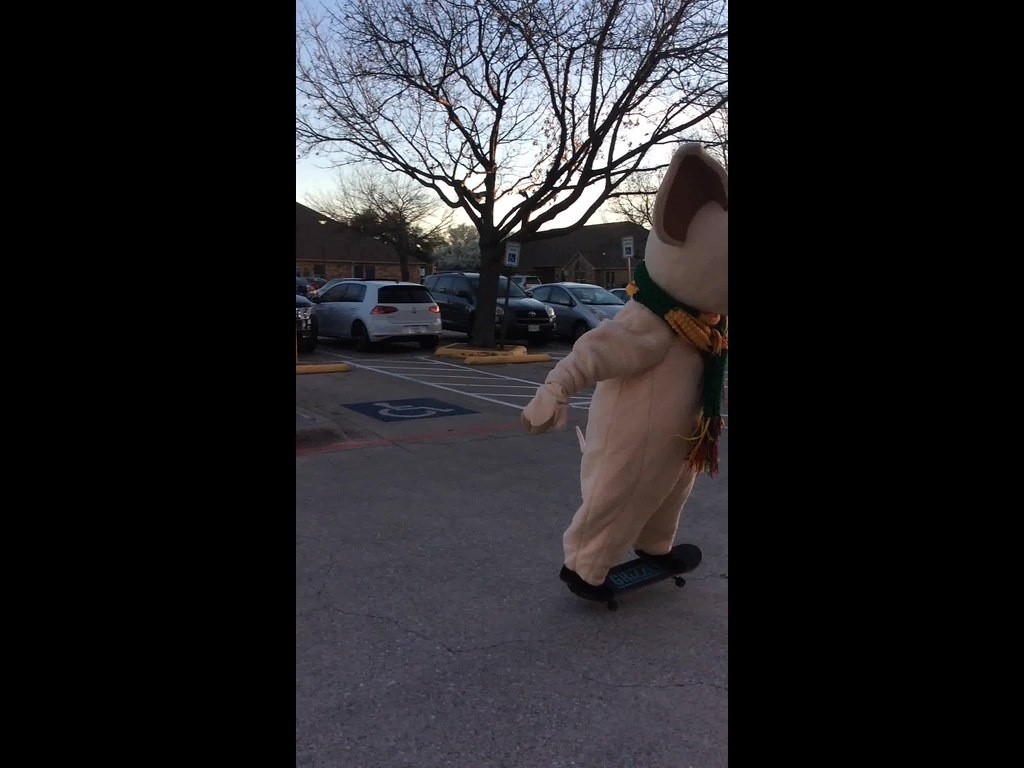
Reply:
[422,272,558,344]
[508,275,541,291]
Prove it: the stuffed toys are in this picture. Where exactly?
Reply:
[520,141,728,600]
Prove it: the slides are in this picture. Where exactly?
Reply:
[568,543,706,610]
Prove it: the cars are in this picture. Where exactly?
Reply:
[306,276,329,290]
[296,293,318,355]
[312,279,443,353]
[608,289,630,304]
[523,282,626,346]
[296,277,313,297]
